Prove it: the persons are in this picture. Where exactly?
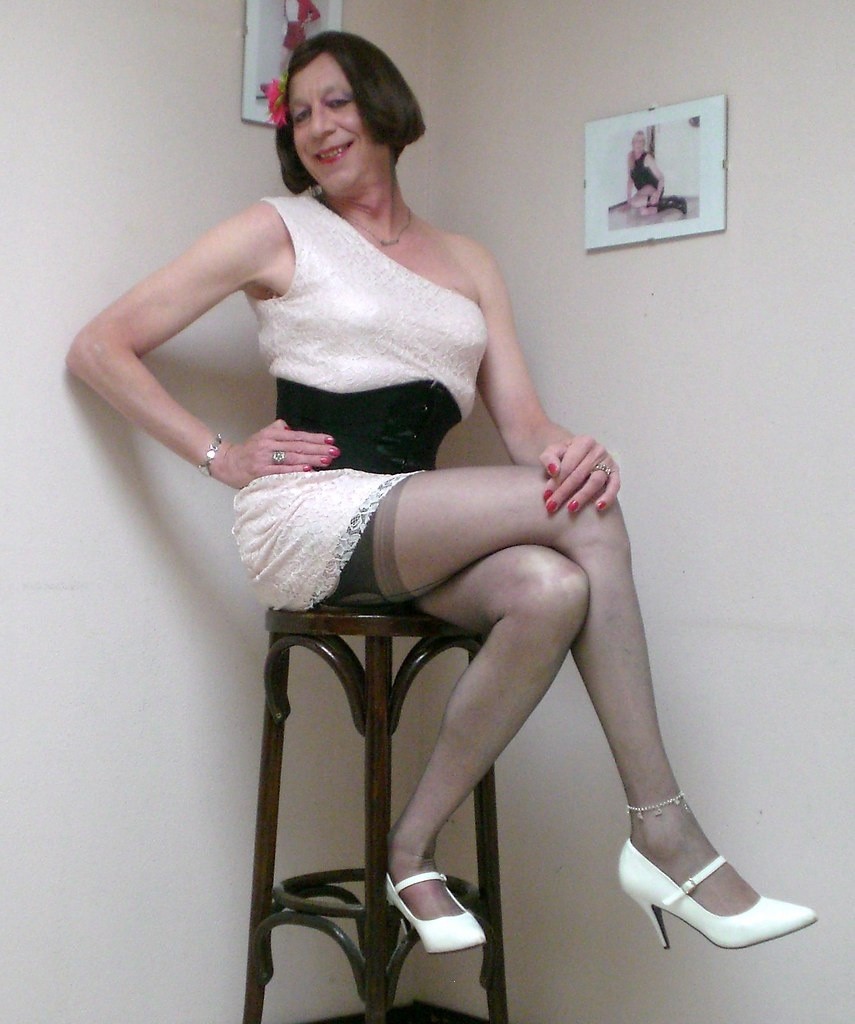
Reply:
[625,131,687,217]
[66,32,821,954]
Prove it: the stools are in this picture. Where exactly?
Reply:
[241,608,511,1024]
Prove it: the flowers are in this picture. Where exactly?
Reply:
[263,68,289,128]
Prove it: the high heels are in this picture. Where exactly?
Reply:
[617,838,817,950]
[386,871,486,954]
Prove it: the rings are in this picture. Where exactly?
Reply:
[594,462,612,476]
[273,449,285,464]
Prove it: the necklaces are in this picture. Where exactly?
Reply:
[329,198,411,245]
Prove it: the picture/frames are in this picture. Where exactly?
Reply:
[240,0,343,128]
[584,93,726,252]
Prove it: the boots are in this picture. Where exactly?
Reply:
[646,196,687,215]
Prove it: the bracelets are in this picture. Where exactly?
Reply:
[196,432,222,475]
